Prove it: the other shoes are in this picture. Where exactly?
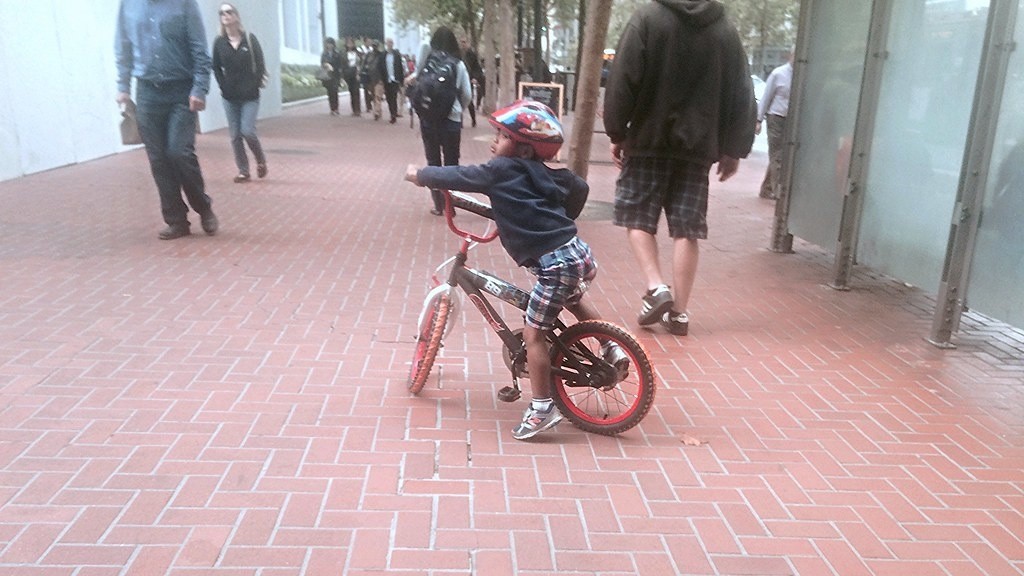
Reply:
[444,207,456,217]
[375,113,378,121]
[760,192,776,199]
[200,199,217,235]
[430,207,442,215]
[258,158,267,177]
[234,172,250,183]
[390,118,396,123]
[159,220,190,239]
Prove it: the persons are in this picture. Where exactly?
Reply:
[404,100,629,438]
[212,3,268,181]
[404,26,472,216]
[460,47,552,128]
[756,46,795,201]
[321,37,417,124]
[604,0,757,334]
[114,0,218,239]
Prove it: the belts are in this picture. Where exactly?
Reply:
[138,78,186,90]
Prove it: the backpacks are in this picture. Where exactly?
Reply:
[408,50,459,122]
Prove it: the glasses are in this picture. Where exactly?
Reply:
[219,10,233,16]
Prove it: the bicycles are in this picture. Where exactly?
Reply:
[403,179,657,435]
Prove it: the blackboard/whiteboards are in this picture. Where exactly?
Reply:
[518,82,563,123]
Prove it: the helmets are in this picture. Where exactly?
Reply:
[489,100,564,160]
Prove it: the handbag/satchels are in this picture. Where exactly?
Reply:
[246,32,270,87]
[121,100,143,144]
[315,67,331,81]
[360,68,370,84]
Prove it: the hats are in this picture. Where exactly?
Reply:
[371,40,379,47]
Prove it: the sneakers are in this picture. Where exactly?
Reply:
[510,402,562,439]
[637,284,674,325]
[659,311,689,336]
[602,344,629,392]
